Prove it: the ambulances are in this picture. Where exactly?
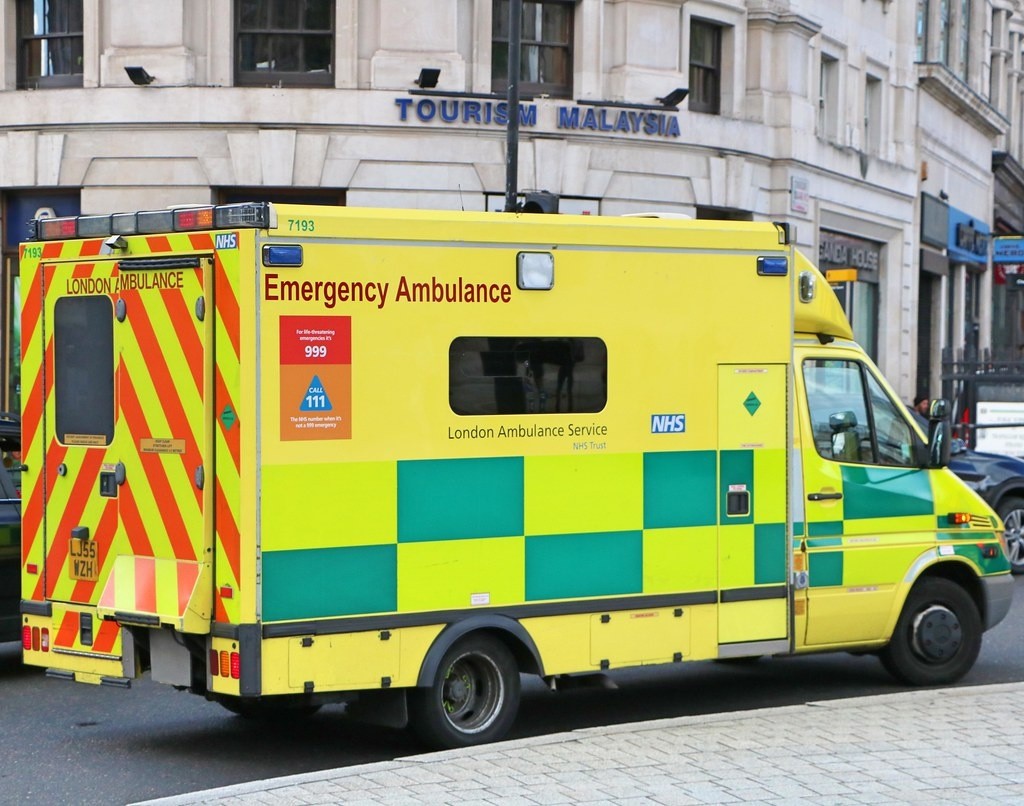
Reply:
[17,200,1018,747]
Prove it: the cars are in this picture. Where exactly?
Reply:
[0,410,23,667]
[808,386,1024,579]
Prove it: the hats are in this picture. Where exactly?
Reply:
[914,397,927,406]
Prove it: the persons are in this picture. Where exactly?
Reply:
[914,396,929,417]
